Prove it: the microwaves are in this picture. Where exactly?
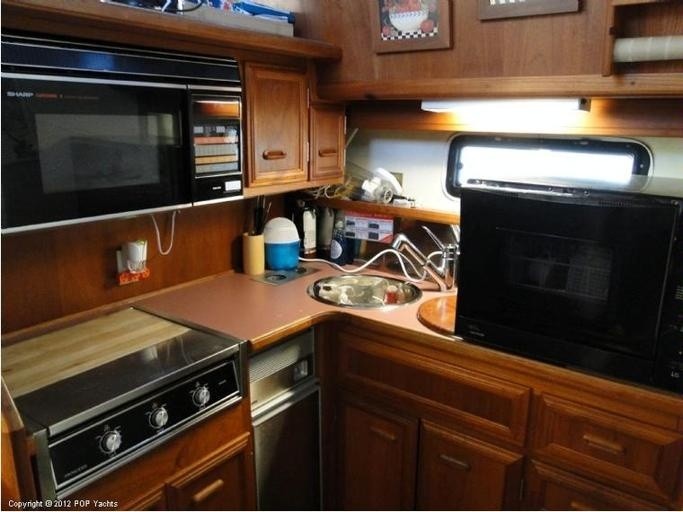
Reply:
[2,28,244,234]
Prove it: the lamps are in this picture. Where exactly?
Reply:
[421,97,592,114]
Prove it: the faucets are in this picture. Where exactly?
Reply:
[390,225,457,291]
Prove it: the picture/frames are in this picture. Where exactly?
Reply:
[478,0,580,21]
[369,0,453,54]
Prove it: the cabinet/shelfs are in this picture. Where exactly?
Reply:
[309,101,347,188]
[327,311,537,510]
[521,362,683,511]
[243,50,310,198]
[59,398,260,511]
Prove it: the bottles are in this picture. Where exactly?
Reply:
[330,217,347,266]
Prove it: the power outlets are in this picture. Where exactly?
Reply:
[108,239,149,284]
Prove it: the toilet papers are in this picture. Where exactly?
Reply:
[612,35,683,64]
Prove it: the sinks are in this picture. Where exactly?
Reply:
[306,273,423,310]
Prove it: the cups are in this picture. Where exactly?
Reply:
[242,231,265,276]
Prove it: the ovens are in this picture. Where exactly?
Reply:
[454,179,683,394]
